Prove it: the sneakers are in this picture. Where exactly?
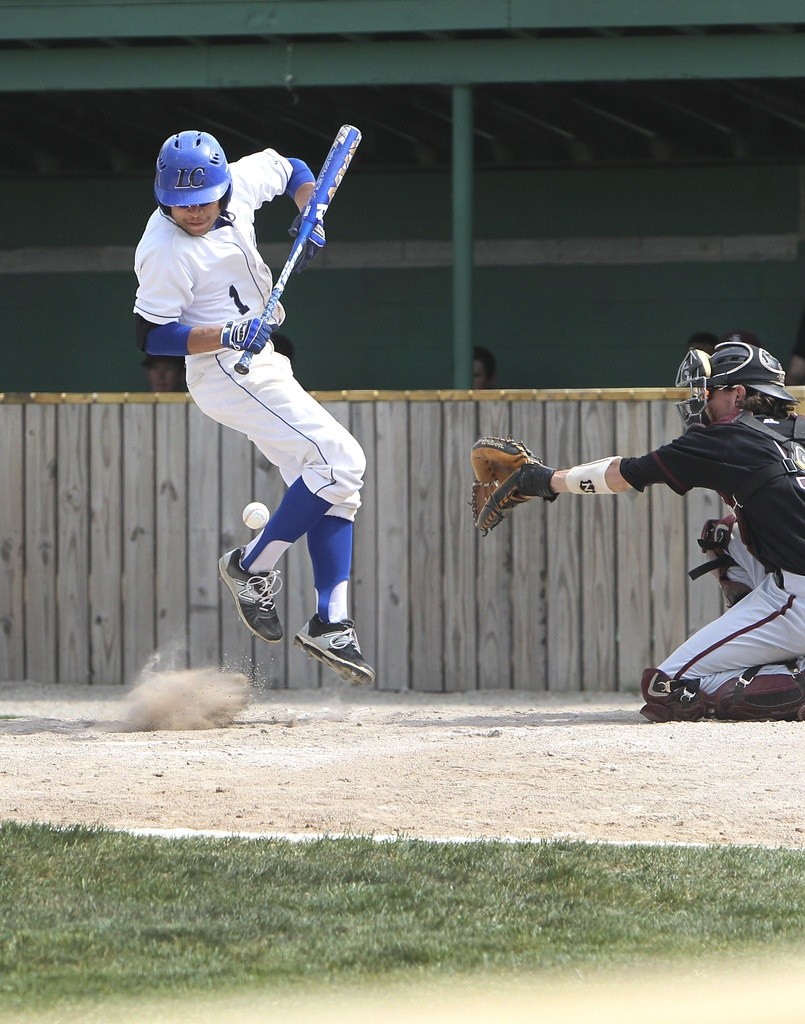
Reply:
[293,612,375,686]
[217,545,284,643]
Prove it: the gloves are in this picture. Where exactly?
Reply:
[289,207,328,274]
[221,318,273,354]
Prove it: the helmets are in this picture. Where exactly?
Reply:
[707,341,800,402]
[154,129,233,217]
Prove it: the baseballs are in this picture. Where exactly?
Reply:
[241,501,271,530]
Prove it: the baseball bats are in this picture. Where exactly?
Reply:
[233,123,363,375]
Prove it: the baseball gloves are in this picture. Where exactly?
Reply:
[468,435,557,537]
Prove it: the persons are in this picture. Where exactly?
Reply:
[132,129,375,685]
[470,338,804,723]
